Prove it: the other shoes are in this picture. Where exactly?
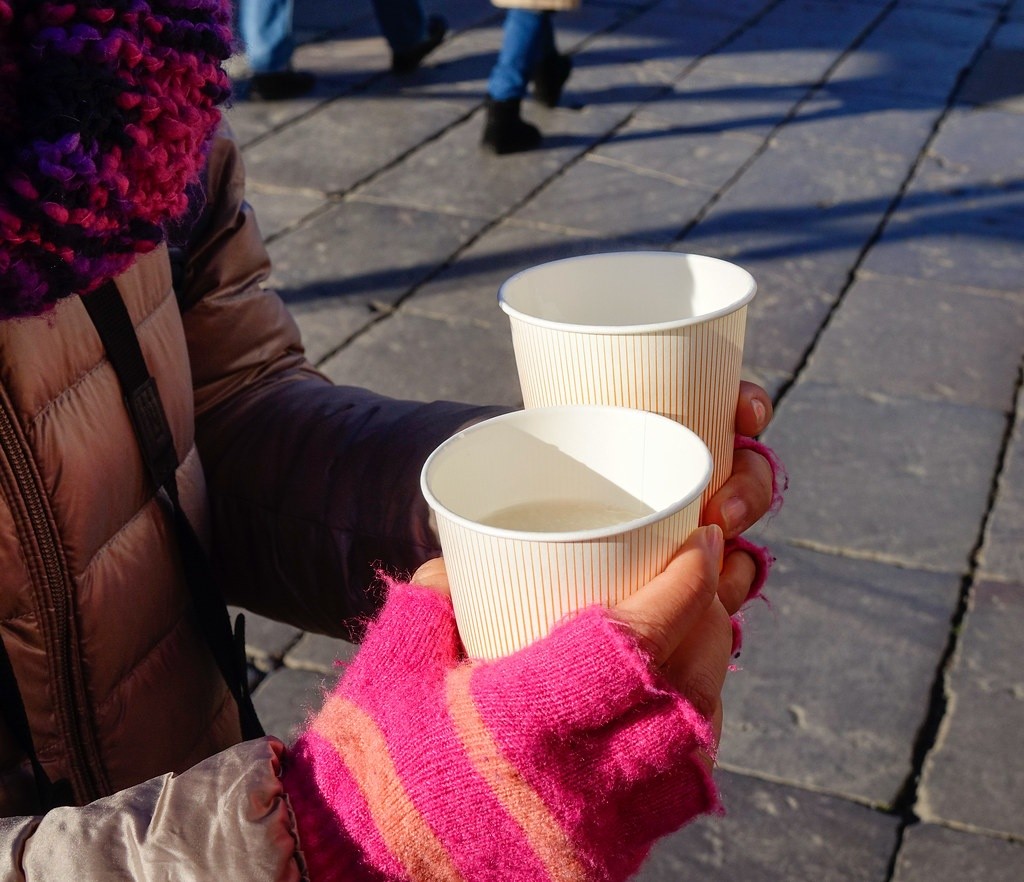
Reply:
[392,13,448,77]
[248,70,316,102]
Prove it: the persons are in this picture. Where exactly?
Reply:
[485,0,582,155]
[238,0,450,99]
[0,0,772,882]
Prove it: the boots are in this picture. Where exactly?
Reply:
[536,56,571,107]
[483,94,542,153]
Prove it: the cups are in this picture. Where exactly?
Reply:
[418,406,714,660]
[497,250,758,527]
